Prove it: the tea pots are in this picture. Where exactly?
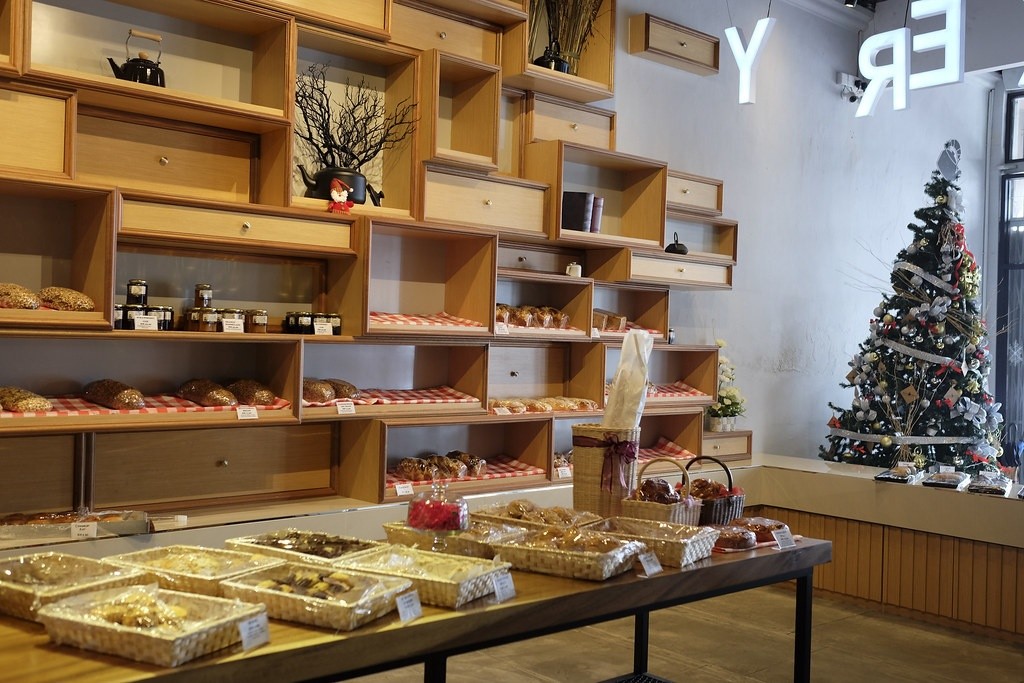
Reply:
[666,232,689,254]
[107,29,165,88]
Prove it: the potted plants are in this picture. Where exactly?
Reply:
[546,0,617,77]
[293,59,423,206]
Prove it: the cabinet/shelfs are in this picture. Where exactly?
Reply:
[0,0,738,545]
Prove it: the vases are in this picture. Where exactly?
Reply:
[709,417,737,433]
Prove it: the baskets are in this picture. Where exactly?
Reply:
[682,455,745,525]
[383,511,528,559]
[620,456,703,529]
[100,544,287,600]
[582,511,720,568]
[226,527,392,568]
[332,544,513,610]
[570,423,641,516]
[36,584,266,666]
[1,551,158,625]
[470,499,601,530]
[217,562,413,632]
[488,528,647,580]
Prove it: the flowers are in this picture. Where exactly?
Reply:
[707,339,748,420]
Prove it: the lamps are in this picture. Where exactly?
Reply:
[845,0,857,8]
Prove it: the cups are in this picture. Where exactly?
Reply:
[565,261,582,277]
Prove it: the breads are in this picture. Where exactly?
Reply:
[488,395,598,413]
[593,307,627,331]
[495,302,571,329]
[630,477,744,506]
[0,282,96,312]
[0,500,638,636]
[0,379,276,414]
[395,450,487,481]
[894,465,1012,492]
[303,376,360,403]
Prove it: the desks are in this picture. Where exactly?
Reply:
[0,533,836,683]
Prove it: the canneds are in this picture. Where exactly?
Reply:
[113,279,341,336]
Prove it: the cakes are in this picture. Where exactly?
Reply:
[696,524,757,549]
[729,517,786,542]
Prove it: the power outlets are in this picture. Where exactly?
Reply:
[836,71,866,92]
